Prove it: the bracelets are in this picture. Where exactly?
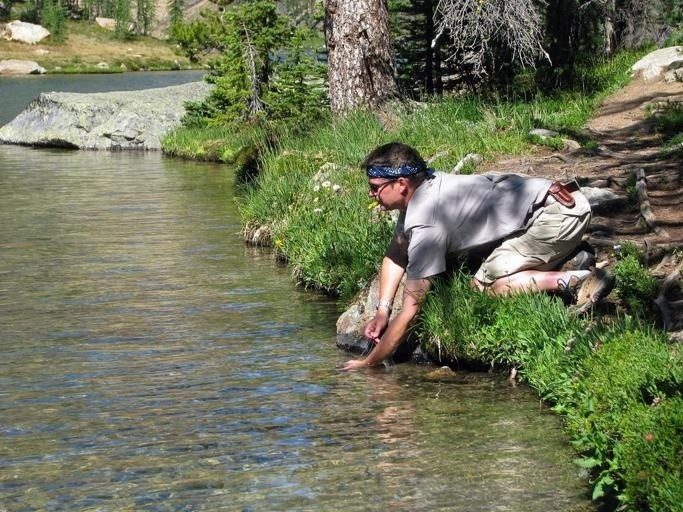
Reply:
[376,299,393,313]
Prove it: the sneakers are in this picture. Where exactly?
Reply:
[553,245,594,271]
[557,267,616,318]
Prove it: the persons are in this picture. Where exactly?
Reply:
[332,141,617,373]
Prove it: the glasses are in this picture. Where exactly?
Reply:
[370,179,397,193]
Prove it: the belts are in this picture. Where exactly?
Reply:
[528,181,580,218]
